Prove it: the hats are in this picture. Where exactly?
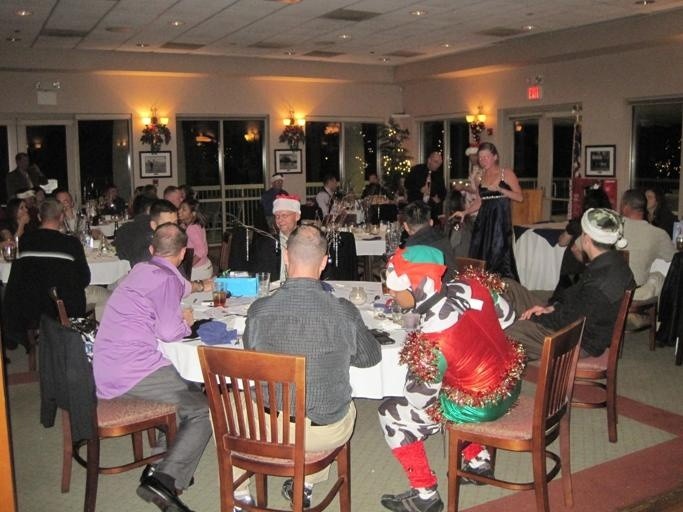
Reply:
[580,207,627,250]
[270,173,283,184]
[271,193,301,214]
[464,144,479,155]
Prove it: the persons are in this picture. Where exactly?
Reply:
[0,156,216,512]
[644,191,675,235]
[378,245,527,512]
[498,209,637,368]
[546,184,613,309]
[209,223,383,512]
[619,188,680,301]
[248,143,525,282]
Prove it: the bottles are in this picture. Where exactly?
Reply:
[124,203,129,220]
[349,287,368,305]
[113,221,118,237]
[386,221,390,233]
[64,226,94,248]
[675,226,683,250]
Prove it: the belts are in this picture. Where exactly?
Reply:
[263,407,319,426]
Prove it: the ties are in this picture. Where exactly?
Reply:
[422,171,431,204]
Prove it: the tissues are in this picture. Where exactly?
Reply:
[213,270,259,297]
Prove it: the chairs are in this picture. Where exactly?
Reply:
[568,284,636,442]
[38,316,178,511]
[455,257,486,272]
[324,231,361,280]
[24,286,97,371]
[228,223,279,279]
[617,297,657,358]
[49,288,157,448]
[217,232,233,275]
[196,342,350,512]
[446,317,586,512]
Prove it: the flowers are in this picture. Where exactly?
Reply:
[276,124,304,149]
[140,123,170,150]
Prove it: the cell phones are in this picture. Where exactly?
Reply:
[376,335,395,344]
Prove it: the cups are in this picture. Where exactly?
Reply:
[255,272,270,296]
[390,299,421,334]
[1,247,17,263]
[209,282,227,307]
[381,282,391,294]
[329,220,384,235]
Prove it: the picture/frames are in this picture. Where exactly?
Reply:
[273,149,302,175]
[584,144,616,178]
[138,151,172,178]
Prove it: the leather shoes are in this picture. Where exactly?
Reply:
[136,475,193,511]
[140,463,194,485]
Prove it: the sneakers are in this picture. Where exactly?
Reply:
[233,490,254,511]
[458,462,493,485]
[381,488,443,511]
[280,478,314,510]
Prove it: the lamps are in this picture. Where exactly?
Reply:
[194,130,212,142]
[514,120,522,133]
[323,122,340,136]
[243,123,259,144]
[465,114,486,145]
[282,109,305,128]
[141,104,168,127]
